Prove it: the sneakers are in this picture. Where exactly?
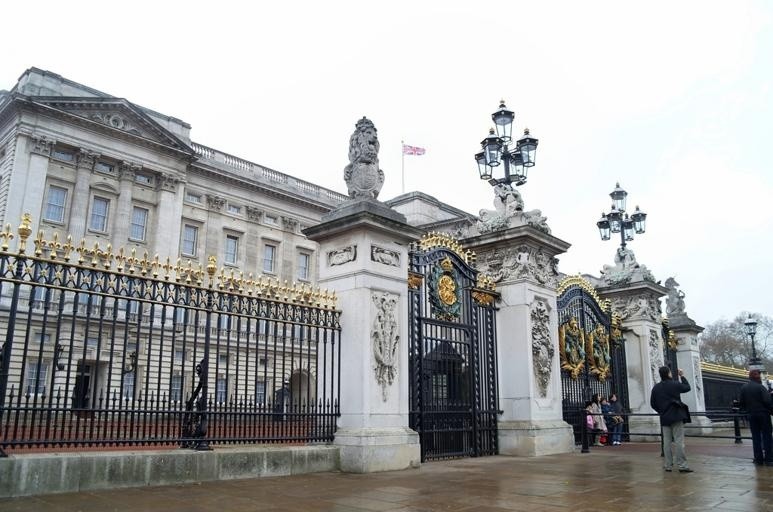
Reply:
[613,441,621,445]
[765,461,773,464]
[666,469,694,472]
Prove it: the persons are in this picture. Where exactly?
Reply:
[650,365,694,473]
[738,368,773,466]
[585,393,625,447]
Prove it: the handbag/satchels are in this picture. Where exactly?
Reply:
[682,402,691,423]
[612,416,624,425]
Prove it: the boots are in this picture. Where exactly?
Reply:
[592,435,604,447]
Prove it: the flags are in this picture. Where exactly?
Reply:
[402,144,426,156]
